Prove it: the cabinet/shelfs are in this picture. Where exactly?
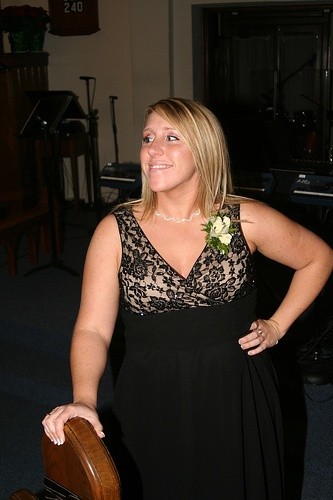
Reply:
[0,52,62,278]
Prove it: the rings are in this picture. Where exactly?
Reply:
[46,413,51,416]
[258,329,264,335]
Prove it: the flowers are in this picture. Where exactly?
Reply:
[200,208,239,256]
[0,6,52,32]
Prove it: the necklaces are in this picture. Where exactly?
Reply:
[154,207,199,224]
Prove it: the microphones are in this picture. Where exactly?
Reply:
[109,96,118,99]
[80,77,95,80]
[310,54,317,66]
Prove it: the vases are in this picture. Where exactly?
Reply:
[9,32,45,52]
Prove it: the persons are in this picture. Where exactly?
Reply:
[42,98,333,500]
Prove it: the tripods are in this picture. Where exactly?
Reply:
[23,90,90,278]
